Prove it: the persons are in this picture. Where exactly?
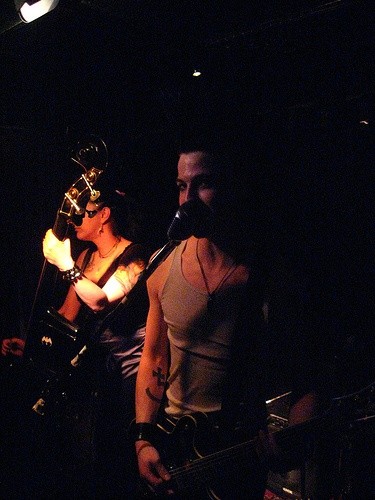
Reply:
[1,184,152,415]
[135,125,321,500]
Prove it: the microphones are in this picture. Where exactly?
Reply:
[166,198,209,242]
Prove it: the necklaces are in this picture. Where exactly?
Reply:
[193,238,236,301]
[96,241,119,258]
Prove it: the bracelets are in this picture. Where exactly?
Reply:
[60,265,82,283]
[134,423,156,441]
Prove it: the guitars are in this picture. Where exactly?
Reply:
[0,164,103,429]
[136,382,375,500]
